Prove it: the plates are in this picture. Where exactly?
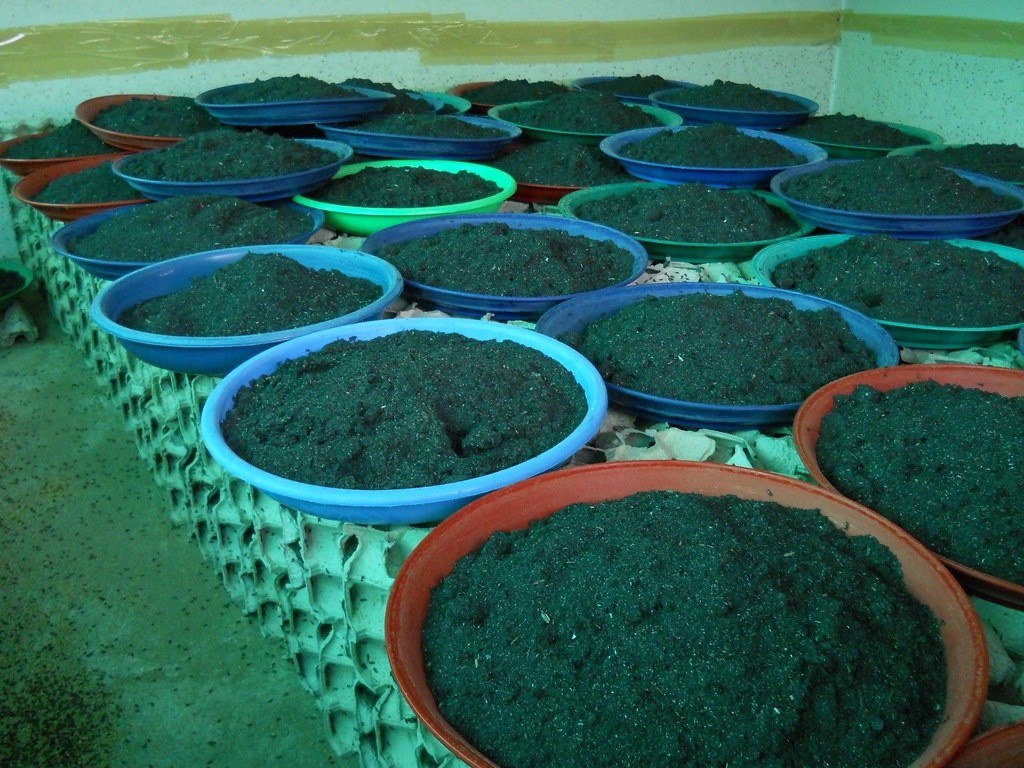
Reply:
[0,76,1024,768]
